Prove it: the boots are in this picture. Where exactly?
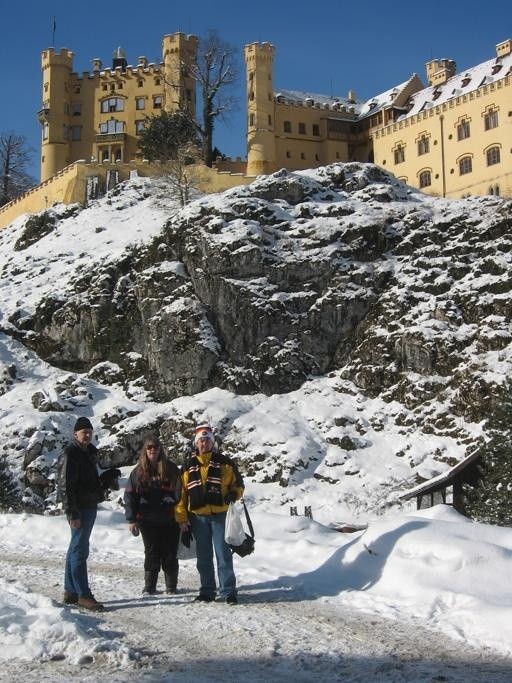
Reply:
[142,570,158,594]
[164,571,177,594]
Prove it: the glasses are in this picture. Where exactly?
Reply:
[146,444,161,450]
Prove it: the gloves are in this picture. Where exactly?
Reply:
[224,492,237,505]
[181,525,193,548]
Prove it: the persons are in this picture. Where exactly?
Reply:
[125,435,184,596]
[54,415,120,607]
[174,422,244,606]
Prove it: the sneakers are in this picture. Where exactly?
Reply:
[64,591,77,604]
[191,595,215,602]
[77,596,103,611]
[224,596,238,605]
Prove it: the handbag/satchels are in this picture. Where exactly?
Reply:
[224,532,255,558]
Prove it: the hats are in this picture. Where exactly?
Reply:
[74,417,93,432]
[195,420,215,446]
